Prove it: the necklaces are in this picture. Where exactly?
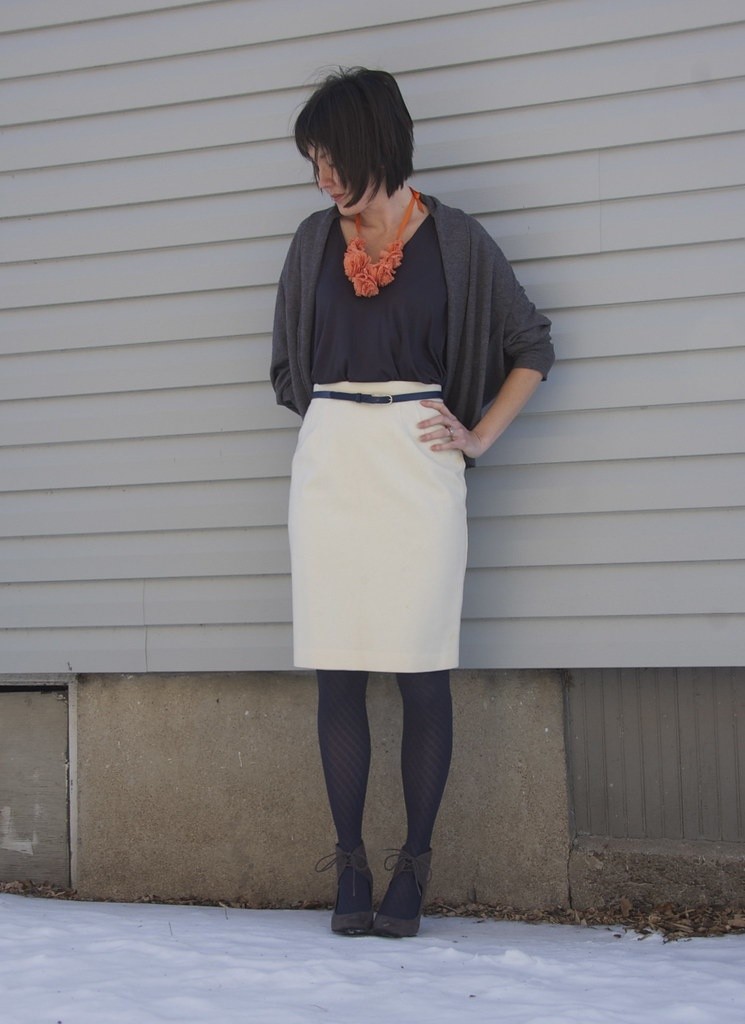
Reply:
[337,190,424,297]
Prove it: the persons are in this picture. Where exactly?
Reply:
[269,65,557,937]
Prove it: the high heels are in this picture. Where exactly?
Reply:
[372,846,433,937]
[315,840,372,935]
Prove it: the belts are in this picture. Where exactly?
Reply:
[313,391,443,405]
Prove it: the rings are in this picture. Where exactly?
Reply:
[447,426,455,438]
